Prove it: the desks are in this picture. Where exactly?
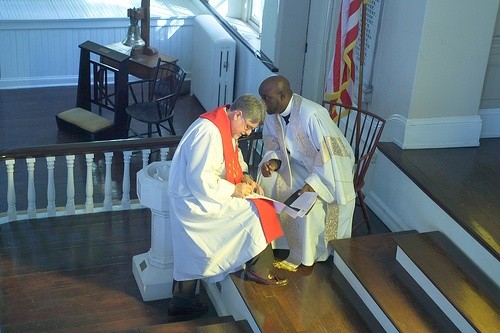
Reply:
[100,42,179,107]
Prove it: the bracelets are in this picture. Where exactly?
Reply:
[243,172,250,176]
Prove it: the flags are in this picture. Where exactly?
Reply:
[323,0,361,122]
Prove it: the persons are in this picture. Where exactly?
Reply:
[167,95,287,318]
[254,76,355,268]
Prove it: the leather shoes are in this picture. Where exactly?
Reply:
[168,300,207,315]
[247,270,287,286]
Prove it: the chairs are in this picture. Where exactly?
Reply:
[55,40,130,140]
[322,100,386,236]
[126,57,186,138]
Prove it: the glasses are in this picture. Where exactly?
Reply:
[243,117,254,130]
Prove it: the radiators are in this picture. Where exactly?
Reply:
[190,13,237,113]
